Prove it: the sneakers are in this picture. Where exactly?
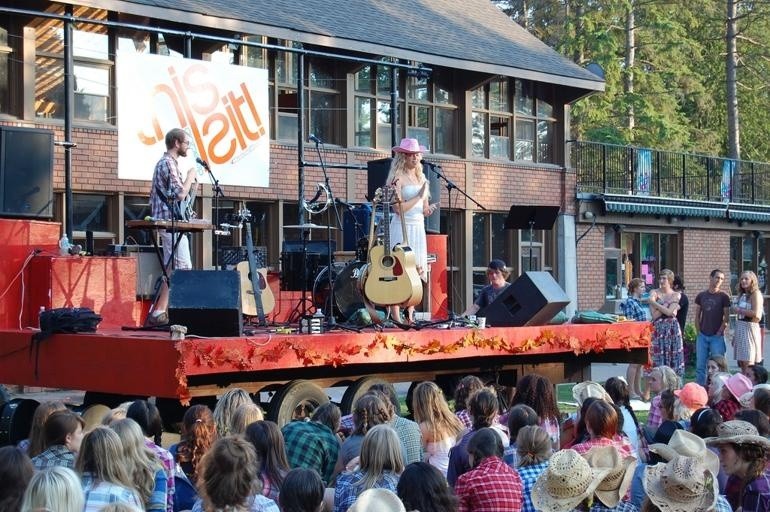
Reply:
[143,312,168,332]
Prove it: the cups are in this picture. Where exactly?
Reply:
[477,317,486,329]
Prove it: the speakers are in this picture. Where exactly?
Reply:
[366,158,440,234]
[0,125,56,221]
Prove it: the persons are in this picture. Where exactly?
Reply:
[18,465,84,511]
[570,398,636,501]
[0,446,35,512]
[447,390,514,485]
[715,375,750,422]
[647,365,681,427]
[733,384,770,436]
[279,403,341,488]
[674,383,708,422]
[643,460,730,512]
[604,377,645,462]
[691,408,723,440]
[454,376,502,432]
[367,390,423,461]
[108,419,168,512]
[325,425,408,512]
[503,404,539,468]
[332,394,407,479]
[625,277,650,400]
[574,398,628,443]
[212,388,253,436]
[463,259,513,318]
[452,428,524,512]
[339,383,401,434]
[192,436,279,512]
[144,128,196,327]
[650,269,685,395]
[650,431,719,483]
[396,462,458,512]
[745,366,766,386]
[81,404,127,433]
[18,400,67,458]
[29,411,84,471]
[224,403,263,437]
[515,424,568,512]
[412,381,468,483]
[73,426,146,512]
[643,388,684,465]
[169,404,220,512]
[493,374,561,453]
[243,421,289,499]
[694,269,729,386]
[704,354,730,403]
[126,401,174,512]
[277,468,326,512]
[732,271,763,375]
[672,276,688,337]
[386,137,433,328]
[706,419,770,512]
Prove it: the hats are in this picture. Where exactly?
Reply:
[704,420,769,448]
[573,381,614,407]
[392,138,426,153]
[673,382,708,410]
[643,456,718,512]
[489,260,506,271]
[719,373,752,402]
[740,384,770,408]
[648,430,720,478]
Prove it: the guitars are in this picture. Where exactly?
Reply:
[363,180,412,304]
[390,177,423,307]
[236,201,276,316]
[173,169,199,222]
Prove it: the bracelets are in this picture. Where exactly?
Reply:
[664,300,672,305]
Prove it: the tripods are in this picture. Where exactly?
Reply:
[503,205,561,270]
[357,307,421,334]
[284,230,326,326]
[316,142,362,334]
[413,167,492,330]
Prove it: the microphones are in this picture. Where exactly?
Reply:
[166,174,171,197]
[196,157,208,169]
[308,134,323,145]
[334,197,352,208]
[420,159,441,168]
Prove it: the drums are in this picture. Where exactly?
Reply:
[334,261,372,313]
[312,264,354,309]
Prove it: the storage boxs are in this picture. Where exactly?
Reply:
[29,252,137,332]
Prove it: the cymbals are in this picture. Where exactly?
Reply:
[281,222,337,231]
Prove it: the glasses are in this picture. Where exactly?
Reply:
[184,141,189,145]
